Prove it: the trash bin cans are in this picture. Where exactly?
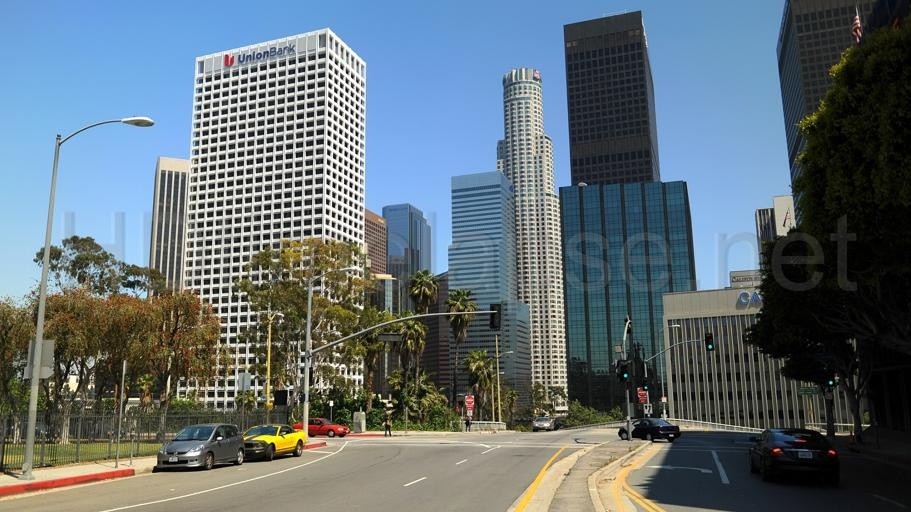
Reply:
[353,412,366,433]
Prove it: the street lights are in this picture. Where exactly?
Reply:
[301,265,367,441]
[495,334,514,422]
[14,115,157,481]
[490,361,505,423]
[265,300,286,426]
[658,324,682,421]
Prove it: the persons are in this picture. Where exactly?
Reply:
[383,411,392,436]
[466,416,471,431]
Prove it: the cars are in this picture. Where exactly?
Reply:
[618,417,682,443]
[746,423,844,487]
[532,417,556,431]
[158,422,245,469]
[11,420,137,440]
[292,417,350,439]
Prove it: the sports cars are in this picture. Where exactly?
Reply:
[241,423,306,461]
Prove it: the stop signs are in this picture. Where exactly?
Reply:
[465,395,475,406]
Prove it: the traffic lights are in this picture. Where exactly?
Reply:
[705,333,715,353]
[827,372,836,388]
[618,361,632,384]
[642,376,649,392]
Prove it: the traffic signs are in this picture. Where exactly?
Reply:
[798,387,819,395]
